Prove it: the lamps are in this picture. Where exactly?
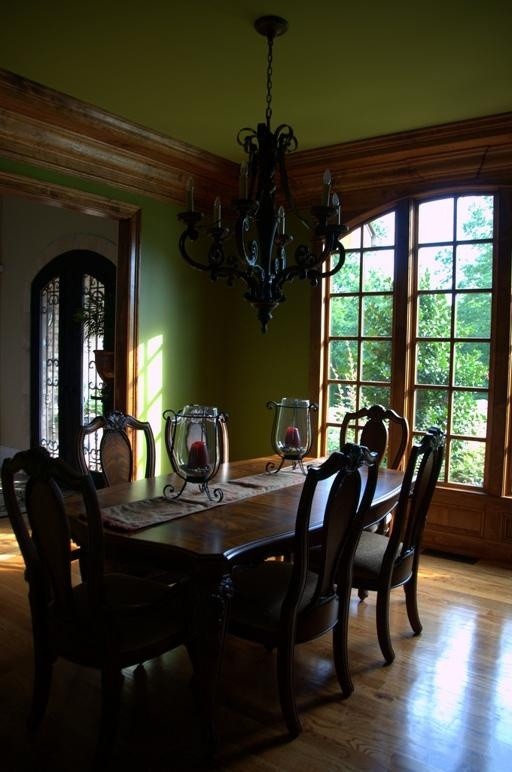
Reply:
[172,14,350,336]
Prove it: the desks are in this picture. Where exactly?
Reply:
[61,451,420,749]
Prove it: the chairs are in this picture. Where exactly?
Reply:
[76,408,157,482]
[2,447,224,723]
[235,441,381,737]
[337,403,410,601]
[307,427,446,662]
